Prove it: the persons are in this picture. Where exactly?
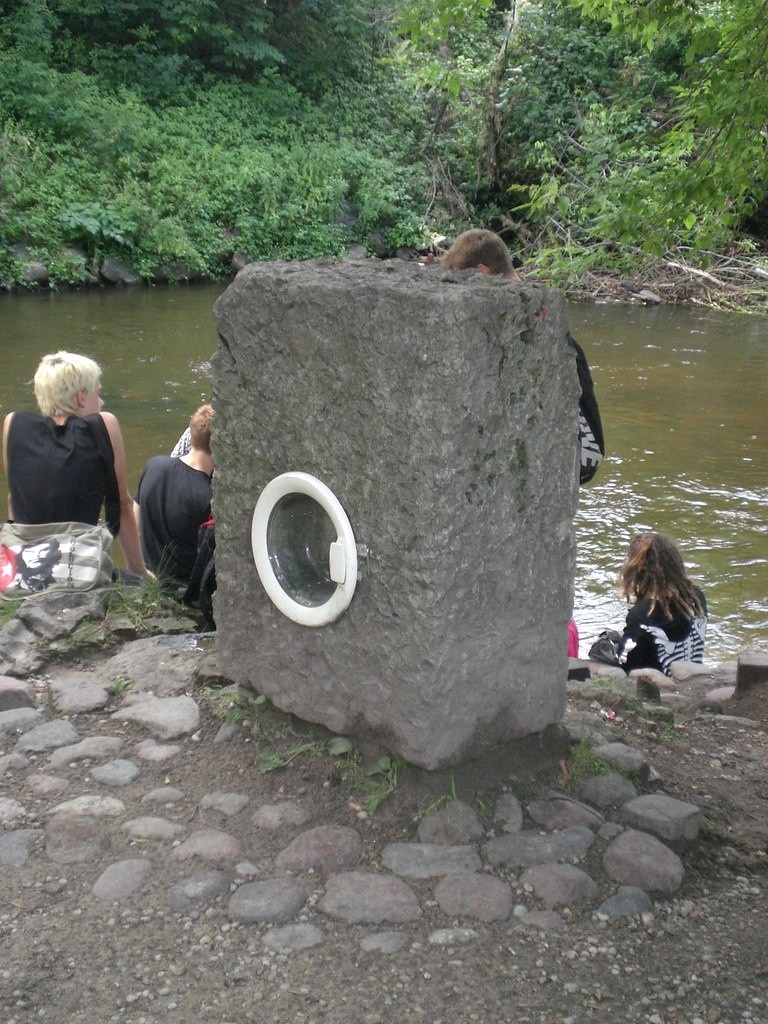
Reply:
[440,228,603,659]
[2,349,158,582]
[587,532,709,678]
[134,402,215,593]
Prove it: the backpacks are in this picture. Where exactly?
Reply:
[178,512,216,631]
[571,337,605,485]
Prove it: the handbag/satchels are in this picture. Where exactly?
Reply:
[0,522,114,602]
[588,630,624,666]
